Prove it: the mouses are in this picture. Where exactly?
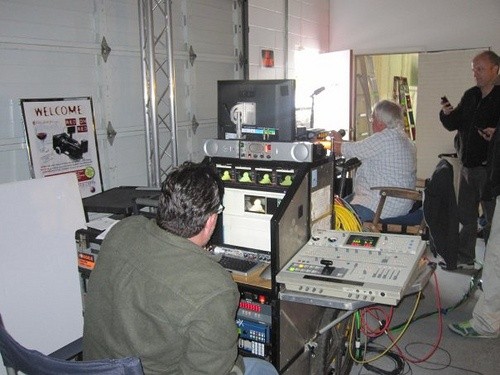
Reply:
[260,264,271,280]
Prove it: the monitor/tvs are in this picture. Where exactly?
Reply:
[217,180,289,255]
[216,79,296,143]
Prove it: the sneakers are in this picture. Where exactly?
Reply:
[448,320,498,338]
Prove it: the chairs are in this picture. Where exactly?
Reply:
[0,313,144,375]
[367,158,453,236]
[333,157,361,203]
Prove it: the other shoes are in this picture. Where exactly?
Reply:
[439,259,475,269]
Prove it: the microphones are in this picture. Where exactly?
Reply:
[311,87,325,97]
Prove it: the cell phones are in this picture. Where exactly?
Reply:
[441,97,451,106]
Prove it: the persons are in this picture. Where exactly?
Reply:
[83,162,278,375]
[331,100,417,222]
[438,51,500,269]
[448,126,500,339]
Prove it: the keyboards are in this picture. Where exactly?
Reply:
[215,256,264,276]
